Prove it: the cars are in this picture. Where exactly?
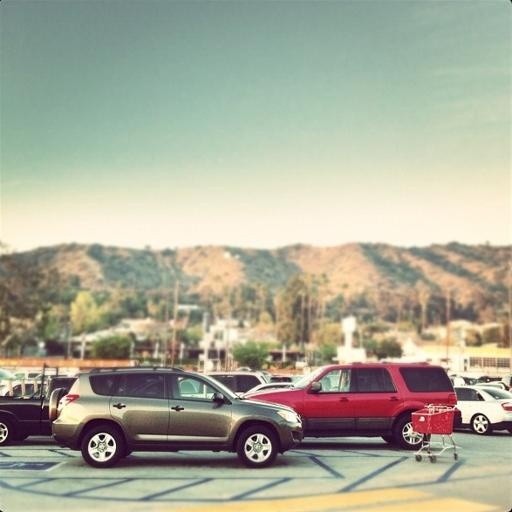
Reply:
[0,363,76,445]
[179,371,268,401]
[454,384,512,436]
[49,365,305,468]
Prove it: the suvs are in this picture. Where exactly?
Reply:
[240,361,458,449]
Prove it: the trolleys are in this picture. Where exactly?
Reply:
[411,405,459,463]
